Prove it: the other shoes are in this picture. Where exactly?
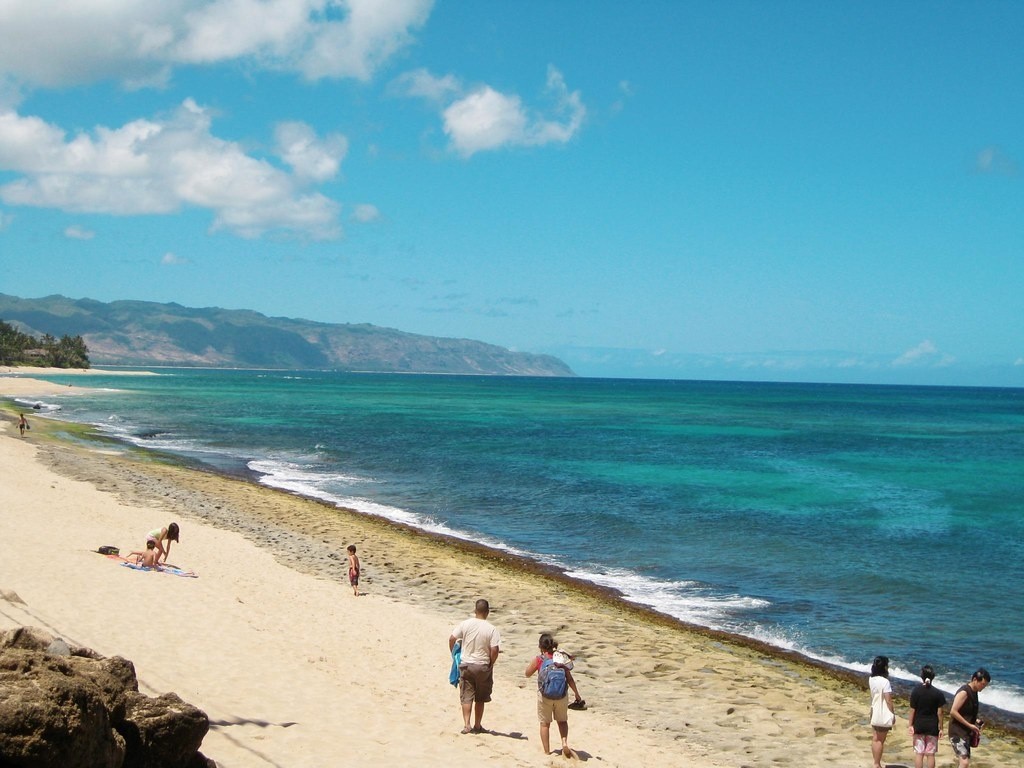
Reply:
[568,700,587,710]
[460,724,488,733]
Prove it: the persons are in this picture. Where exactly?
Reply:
[347,545,360,597]
[525,633,582,759]
[948,669,991,768]
[868,656,896,768]
[16,413,28,438]
[125,522,179,571]
[449,599,501,734]
[906,665,948,768]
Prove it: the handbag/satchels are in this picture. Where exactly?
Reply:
[871,708,894,727]
[970,732,979,747]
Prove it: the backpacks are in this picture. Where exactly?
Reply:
[99,546,119,555]
[537,655,569,700]
